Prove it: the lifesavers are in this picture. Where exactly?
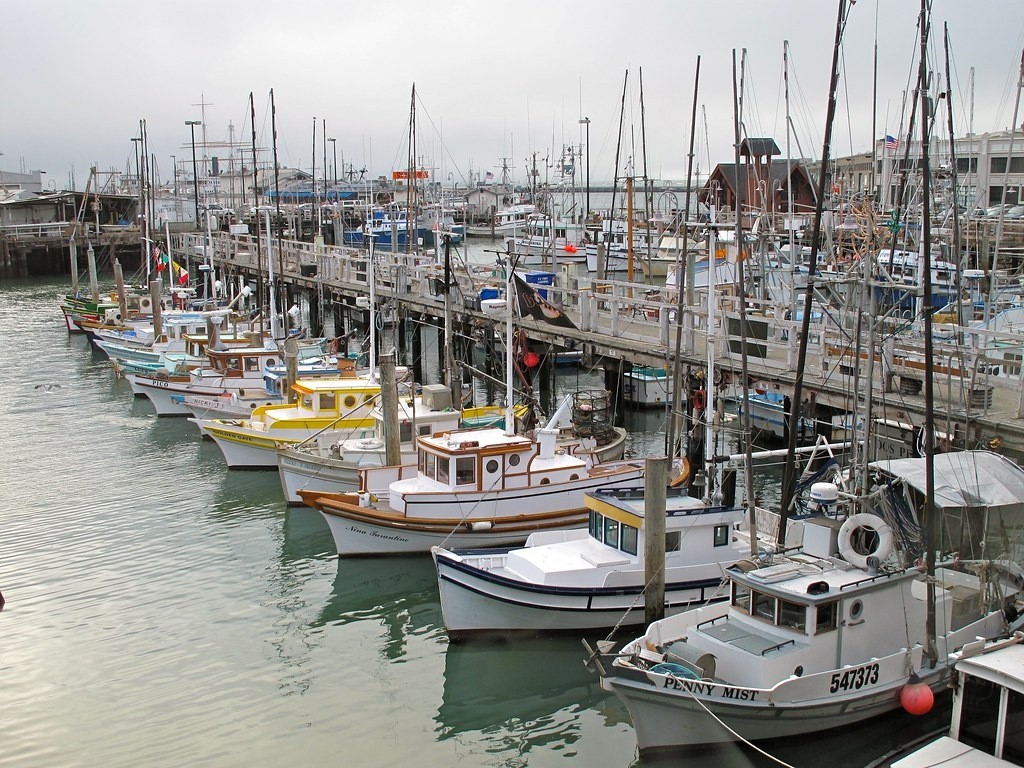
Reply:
[839,512,893,570]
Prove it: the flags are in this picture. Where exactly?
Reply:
[512,273,580,330]
[154,246,169,271]
[486,171,494,180]
[886,135,902,151]
[172,259,189,285]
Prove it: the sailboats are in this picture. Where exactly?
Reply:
[40,0,1024,768]
